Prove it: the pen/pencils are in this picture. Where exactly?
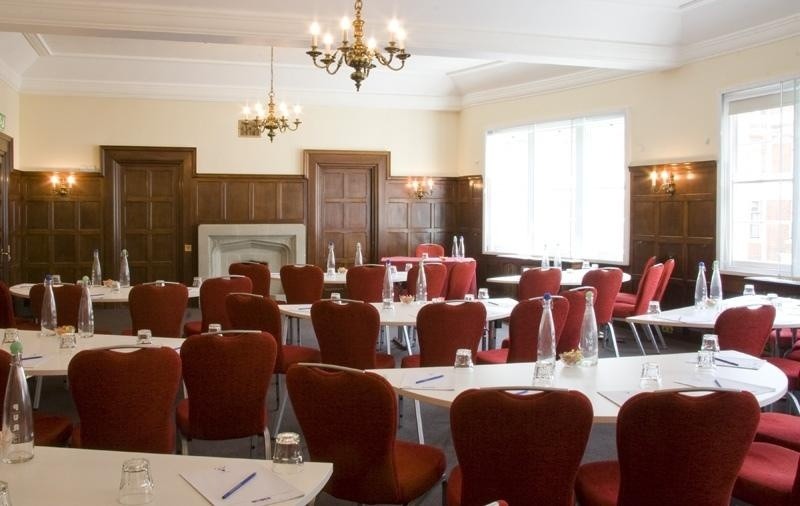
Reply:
[415,375,444,383]
[22,356,42,360]
[516,390,528,394]
[715,379,722,388]
[222,472,257,499]
[715,358,738,366]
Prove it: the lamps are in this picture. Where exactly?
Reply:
[242,46,302,143]
[52,175,74,197]
[305,0,410,93]
[649,170,675,194]
[412,180,433,200]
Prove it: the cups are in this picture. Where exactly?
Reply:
[463,293,475,305]
[700,330,720,355]
[403,262,414,272]
[0,481,12,506]
[476,286,492,303]
[330,292,342,305]
[532,360,560,385]
[579,258,592,271]
[634,358,663,391]
[636,360,665,391]
[0,276,225,350]
[646,297,664,321]
[766,292,779,306]
[739,282,761,298]
[419,250,431,261]
[329,268,339,281]
[117,457,156,506]
[537,249,551,270]
[553,249,563,269]
[270,431,307,484]
[448,347,477,378]
[693,348,717,375]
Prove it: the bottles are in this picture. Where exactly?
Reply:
[0,341,35,466]
[448,234,458,260]
[693,260,707,310]
[415,260,430,308]
[40,250,130,337]
[380,259,395,312]
[536,295,558,366]
[575,289,602,365]
[328,243,337,266]
[459,233,467,258]
[711,259,721,299]
[352,241,365,267]
